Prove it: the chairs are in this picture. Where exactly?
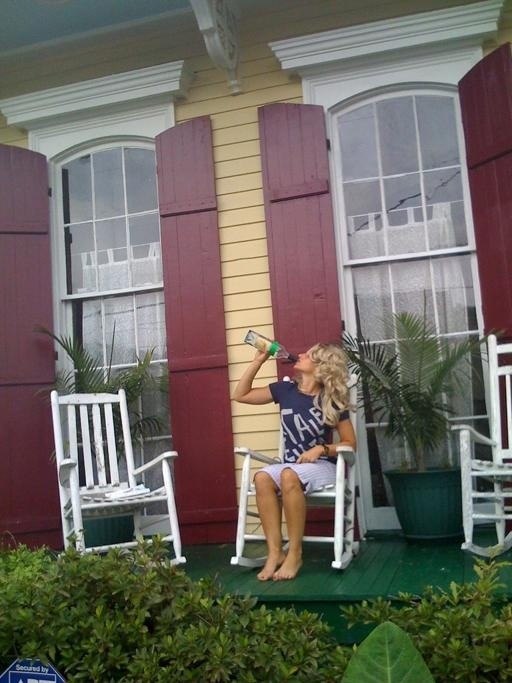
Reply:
[449,333,512,559]
[228,372,360,570]
[48,386,188,568]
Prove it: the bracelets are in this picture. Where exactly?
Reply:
[321,442,330,458]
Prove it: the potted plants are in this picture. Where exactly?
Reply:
[36,313,170,547]
[341,313,502,541]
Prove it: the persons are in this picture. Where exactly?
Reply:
[234,340,358,583]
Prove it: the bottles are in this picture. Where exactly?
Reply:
[243,328,299,362]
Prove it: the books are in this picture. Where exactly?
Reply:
[104,483,151,499]
